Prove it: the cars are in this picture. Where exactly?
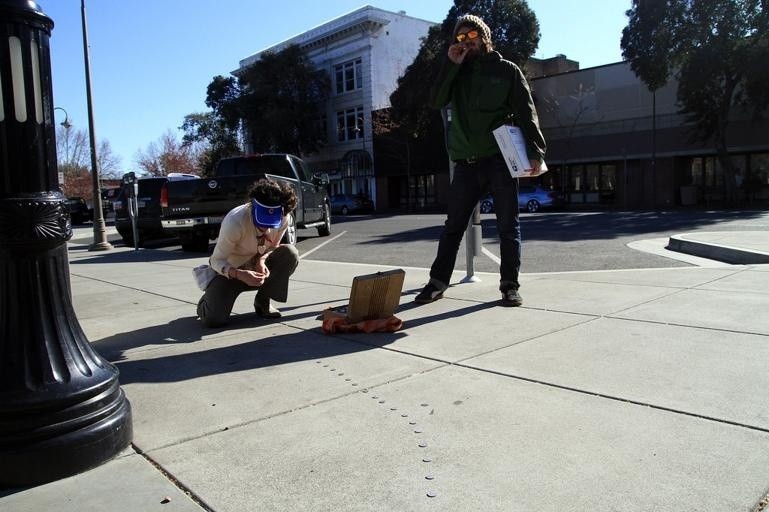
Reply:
[68,153,331,252]
[480,179,565,214]
[331,193,371,215]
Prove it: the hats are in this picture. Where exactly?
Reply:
[452,13,492,44]
[250,196,283,230]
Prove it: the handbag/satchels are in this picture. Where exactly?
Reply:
[191,265,217,291]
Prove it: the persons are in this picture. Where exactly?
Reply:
[191,178,300,328]
[415,12,547,307]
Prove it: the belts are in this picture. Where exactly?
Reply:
[455,156,484,166]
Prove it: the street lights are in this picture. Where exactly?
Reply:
[54,106,73,128]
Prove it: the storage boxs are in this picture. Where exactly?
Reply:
[492,124,549,178]
[322,268,405,325]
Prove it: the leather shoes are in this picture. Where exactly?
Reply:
[253,293,282,319]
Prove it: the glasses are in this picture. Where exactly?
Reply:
[452,29,479,43]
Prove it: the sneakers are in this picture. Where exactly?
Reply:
[414,281,445,305]
[501,289,523,307]
